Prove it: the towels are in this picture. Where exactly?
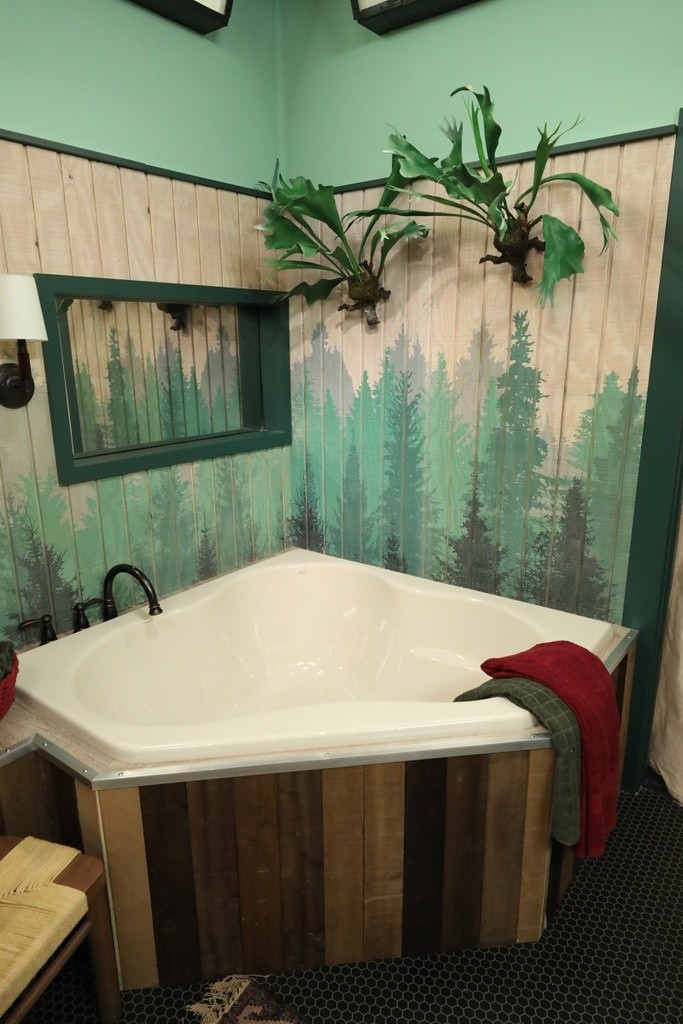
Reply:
[452,639,622,858]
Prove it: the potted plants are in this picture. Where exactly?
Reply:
[258,79,620,326]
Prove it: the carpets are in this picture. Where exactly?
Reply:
[181,970,308,1024]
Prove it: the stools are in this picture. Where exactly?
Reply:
[0,833,122,1024]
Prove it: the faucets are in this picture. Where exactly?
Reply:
[102,563,163,622]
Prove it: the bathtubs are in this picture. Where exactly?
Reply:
[12,544,644,997]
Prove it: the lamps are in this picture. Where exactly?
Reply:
[0,274,49,408]
